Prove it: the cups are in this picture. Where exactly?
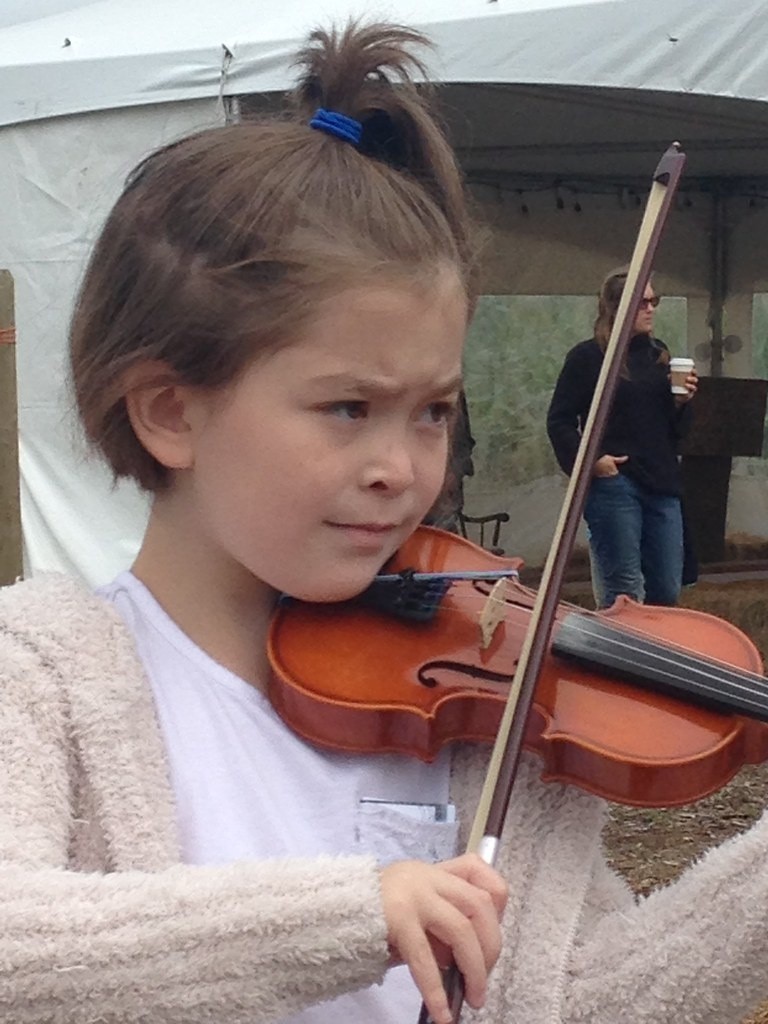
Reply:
[670,357,695,394]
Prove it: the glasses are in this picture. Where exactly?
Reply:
[639,297,660,309]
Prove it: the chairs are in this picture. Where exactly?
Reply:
[458,512,509,558]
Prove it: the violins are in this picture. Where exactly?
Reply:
[261,519,768,811]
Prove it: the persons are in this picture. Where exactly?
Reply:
[545,272,699,611]
[420,384,476,535]
[0,23,768,1024]
